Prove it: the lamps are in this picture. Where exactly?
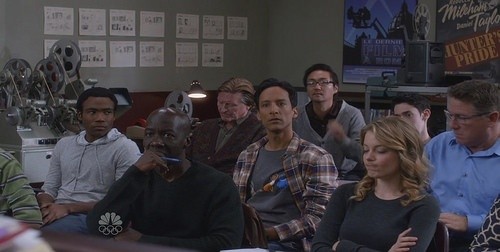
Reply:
[187,80,207,99]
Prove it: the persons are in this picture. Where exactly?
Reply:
[231,78,340,252]
[0,147,54,252]
[33,87,141,236]
[85,109,244,252]
[311,116,440,252]
[424,79,500,252]
[184,79,267,173]
[292,64,365,174]
[391,94,431,145]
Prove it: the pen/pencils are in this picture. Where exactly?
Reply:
[136,154,181,162]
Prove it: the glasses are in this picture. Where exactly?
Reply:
[306,80,334,87]
[444,110,489,121]
